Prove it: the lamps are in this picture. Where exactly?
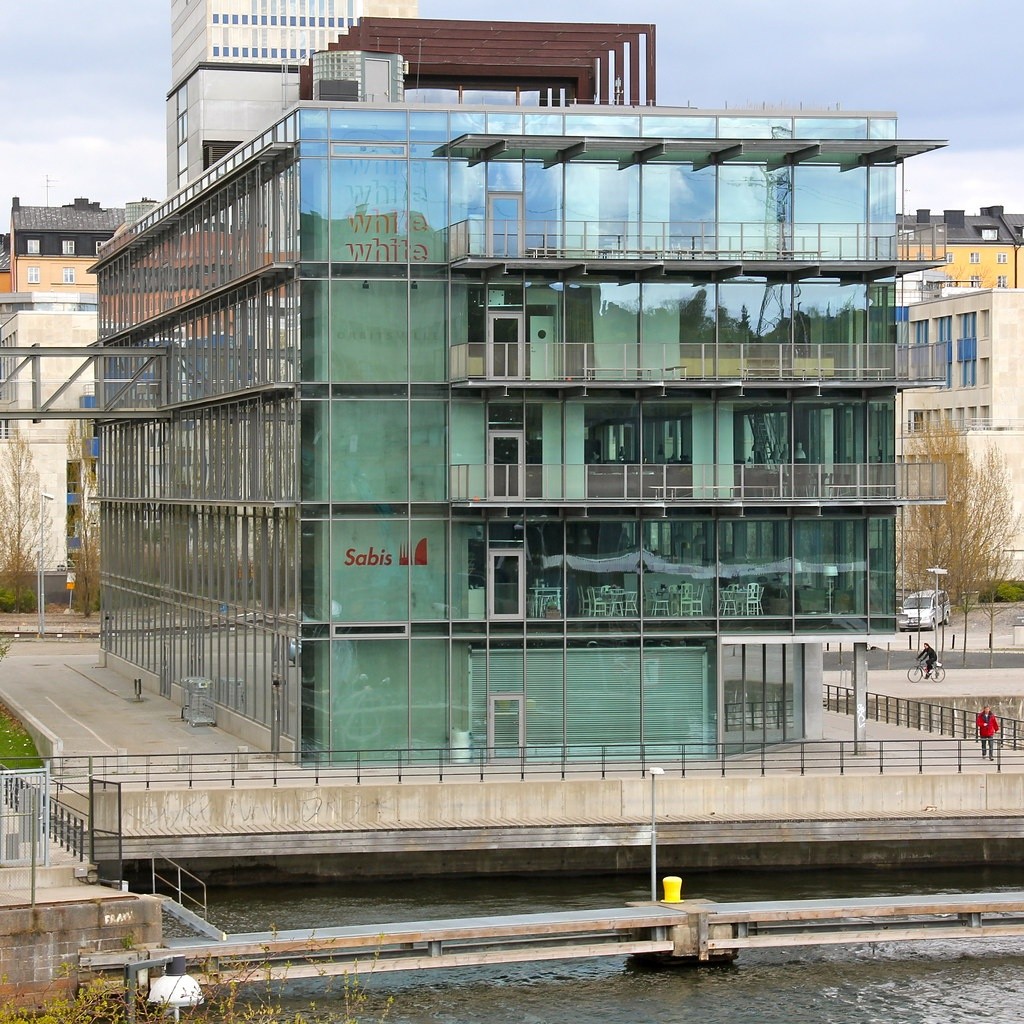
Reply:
[770,413,780,459]
[795,403,807,459]
[780,412,789,459]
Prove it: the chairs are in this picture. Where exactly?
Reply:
[576,583,765,616]
[769,472,853,497]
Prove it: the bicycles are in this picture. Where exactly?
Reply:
[905,656,945,683]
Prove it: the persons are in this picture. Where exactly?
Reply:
[977,705,998,761]
[916,643,937,679]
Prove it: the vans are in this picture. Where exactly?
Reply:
[899,589,951,633]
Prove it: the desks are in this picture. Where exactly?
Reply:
[720,590,762,616]
[595,589,636,616]
[530,587,568,617]
[655,590,696,616]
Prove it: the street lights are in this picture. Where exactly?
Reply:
[927,568,946,660]
[648,767,664,901]
[37,494,53,638]
[122,954,204,1024]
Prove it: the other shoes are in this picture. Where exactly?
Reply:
[925,676,929,679]
[983,755,986,759]
[990,757,994,760]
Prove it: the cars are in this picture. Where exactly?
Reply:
[289,636,302,666]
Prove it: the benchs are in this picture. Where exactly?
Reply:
[528,247,829,260]
[737,368,813,381]
[582,367,659,381]
[650,485,786,500]
[815,367,891,380]
[662,366,687,380]
